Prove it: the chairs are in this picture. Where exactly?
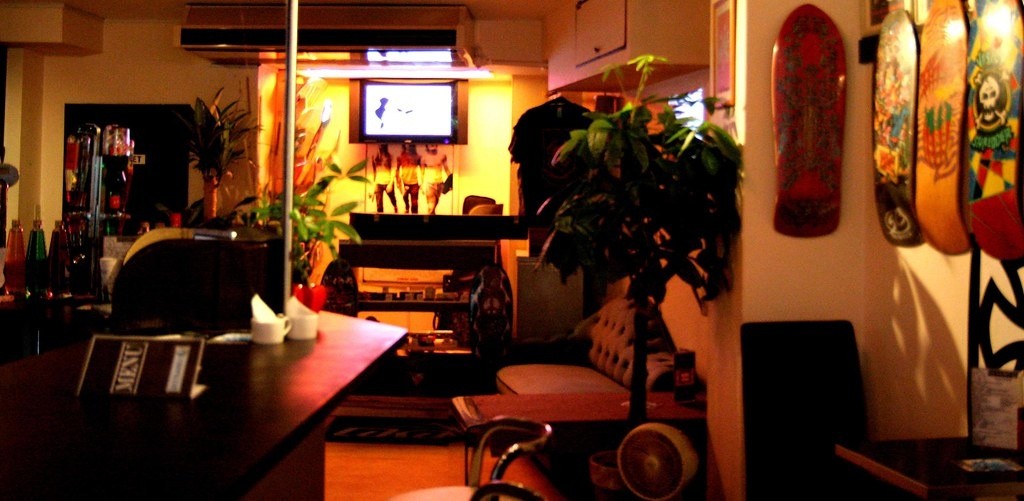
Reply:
[390,421,553,501]
[739,321,874,501]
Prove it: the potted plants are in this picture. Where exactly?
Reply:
[162,90,258,216]
[252,159,370,339]
[514,64,740,501]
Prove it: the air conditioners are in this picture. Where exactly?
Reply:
[171,0,472,65]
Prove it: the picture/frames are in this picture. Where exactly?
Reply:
[709,0,737,109]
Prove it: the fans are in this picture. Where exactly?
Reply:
[616,421,698,501]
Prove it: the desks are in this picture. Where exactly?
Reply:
[451,389,708,486]
[0,316,406,501]
[834,435,1024,501]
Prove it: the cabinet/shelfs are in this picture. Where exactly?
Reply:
[324,238,502,432]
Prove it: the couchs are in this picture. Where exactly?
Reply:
[495,296,705,476]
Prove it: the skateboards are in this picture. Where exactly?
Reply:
[961,0,1024,262]
[769,3,848,239]
[870,8,921,247]
[913,0,971,255]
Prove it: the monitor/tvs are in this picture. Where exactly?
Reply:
[349,78,467,145]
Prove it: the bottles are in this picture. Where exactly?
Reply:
[2,220,26,292]
[102,123,130,209]
[98,217,121,303]
[63,123,97,209]
[25,220,48,294]
[47,220,70,295]
[61,210,90,296]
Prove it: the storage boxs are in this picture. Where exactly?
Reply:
[356,265,453,303]
[356,311,439,345]
[515,254,585,335]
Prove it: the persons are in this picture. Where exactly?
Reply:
[397,141,421,214]
[421,142,453,215]
[372,143,398,212]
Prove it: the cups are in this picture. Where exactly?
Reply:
[285,314,320,340]
[252,316,292,345]
